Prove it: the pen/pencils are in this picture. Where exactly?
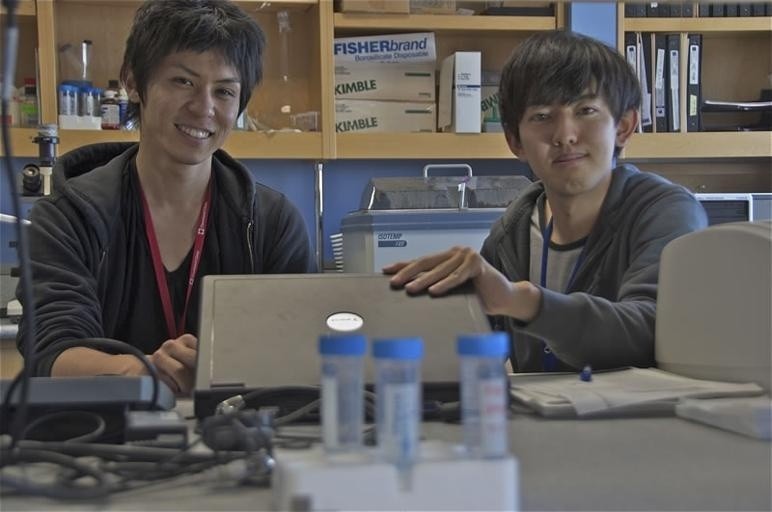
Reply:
[579,365,592,381]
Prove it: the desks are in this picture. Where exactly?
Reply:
[2,354,772,512]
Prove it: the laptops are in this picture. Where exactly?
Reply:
[195,271,495,417]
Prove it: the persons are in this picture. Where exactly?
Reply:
[380,25,709,372]
[16,0,318,400]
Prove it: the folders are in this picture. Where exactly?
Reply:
[623,33,705,134]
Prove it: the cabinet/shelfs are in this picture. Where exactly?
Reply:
[3,1,771,164]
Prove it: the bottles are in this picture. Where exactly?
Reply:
[0,77,128,130]
[317,333,510,468]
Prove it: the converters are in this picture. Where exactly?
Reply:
[201,409,268,452]
[125,409,188,451]
